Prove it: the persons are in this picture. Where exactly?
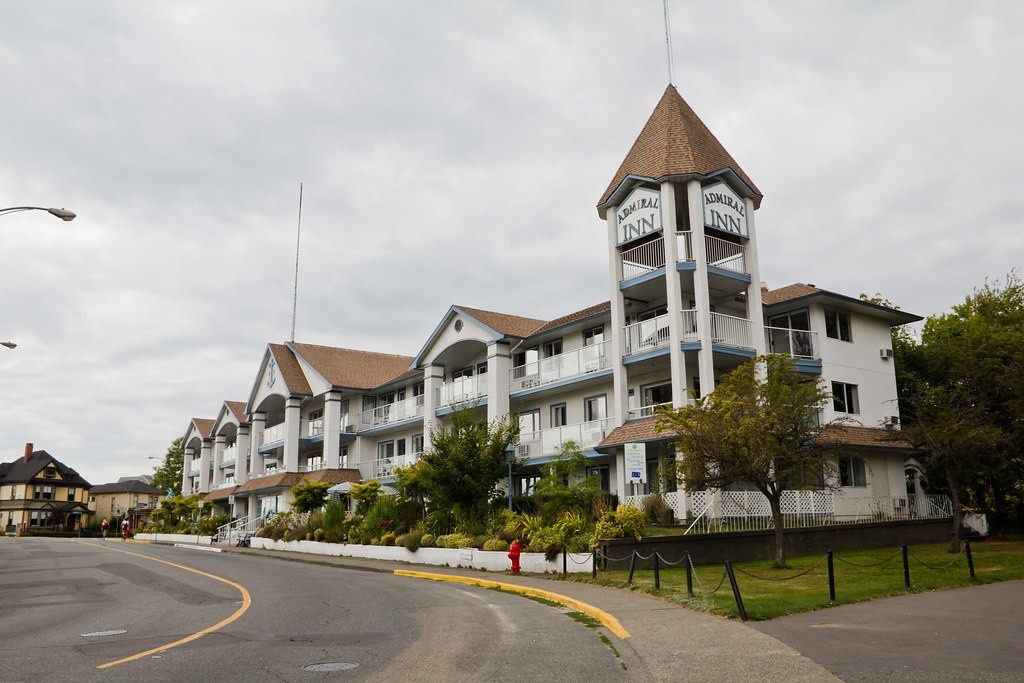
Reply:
[101,518,110,541]
[120,517,130,542]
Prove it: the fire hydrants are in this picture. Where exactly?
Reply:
[506,541,522,575]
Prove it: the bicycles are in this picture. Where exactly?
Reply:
[102,528,108,541]
[122,529,126,541]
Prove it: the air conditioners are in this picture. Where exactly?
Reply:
[880,348,892,359]
[346,424,357,433]
[885,416,898,425]
